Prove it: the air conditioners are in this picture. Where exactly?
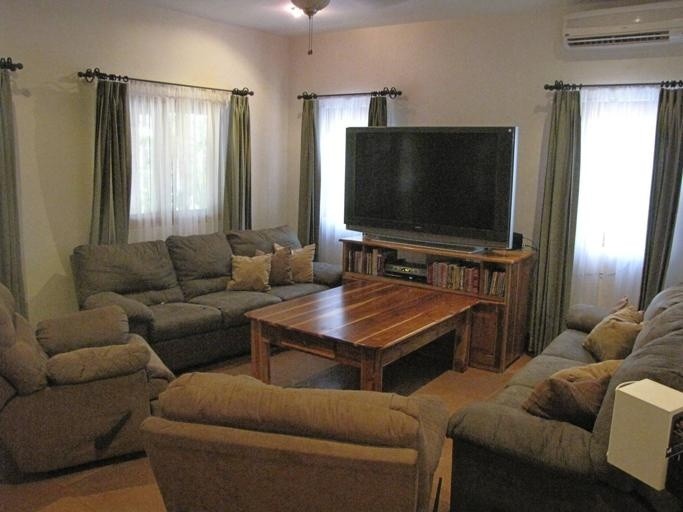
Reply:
[562,1,683,51]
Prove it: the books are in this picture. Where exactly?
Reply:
[347,246,504,299]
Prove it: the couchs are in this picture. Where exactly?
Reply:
[445,282,683,511]
[74,225,343,372]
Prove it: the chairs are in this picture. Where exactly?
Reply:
[140,371,450,512]
[0,281,176,473]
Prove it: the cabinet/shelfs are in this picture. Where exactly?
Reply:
[338,237,539,373]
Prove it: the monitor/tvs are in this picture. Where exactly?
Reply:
[344,126,519,253]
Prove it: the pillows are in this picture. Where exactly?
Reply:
[272,241,316,282]
[582,315,650,362]
[255,246,294,286]
[521,359,625,430]
[227,252,272,295]
[613,304,644,324]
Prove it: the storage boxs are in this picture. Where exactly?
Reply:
[470,307,502,366]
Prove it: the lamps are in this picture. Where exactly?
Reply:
[291,0,331,54]
